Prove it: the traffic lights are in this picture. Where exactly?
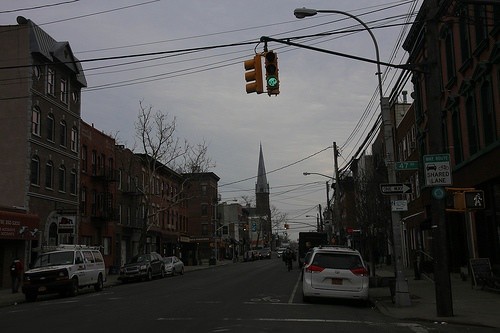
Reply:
[463,190,485,210]
[244,54,263,95]
[264,51,280,95]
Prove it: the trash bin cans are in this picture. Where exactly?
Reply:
[389,279,409,304]
[210,257,216,265]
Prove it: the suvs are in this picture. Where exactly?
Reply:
[21,244,107,303]
[117,252,167,284]
[299,245,371,305]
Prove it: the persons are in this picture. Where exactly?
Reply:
[283,247,295,271]
[11,258,23,294]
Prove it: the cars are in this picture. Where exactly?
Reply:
[162,256,185,277]
[242,248,272,261]
[277,244,291,258]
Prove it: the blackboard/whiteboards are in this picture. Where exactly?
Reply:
[470,258,494,290]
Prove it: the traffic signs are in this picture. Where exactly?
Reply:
[380,183,414,195]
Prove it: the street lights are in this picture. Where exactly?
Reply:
[293,6,412,308]
[303,172,345,245]
